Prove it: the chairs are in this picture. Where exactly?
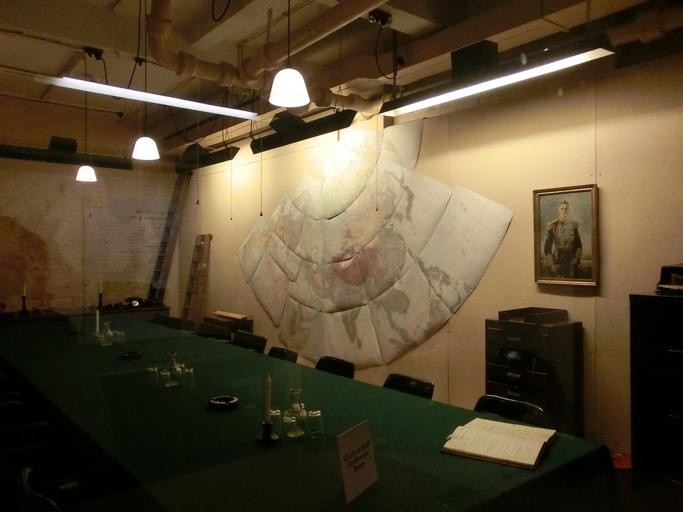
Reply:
[200,322,227,340]
[315,356,355,378]
[232,328,267,353]
[383,373,434,400]
[473,395,544,425]
[168,317,197,331]
[268,347,299,362]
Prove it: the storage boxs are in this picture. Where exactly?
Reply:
[660,263,683,286]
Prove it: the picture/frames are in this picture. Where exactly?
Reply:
[534,184,597,286]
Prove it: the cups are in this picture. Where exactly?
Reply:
[118,332,125,345]
[269,410,282,440]
[184,368,197,387]
[284,418,300,446]
[176,361,184,371]
[156,369,170,391]
[96,334,105,352]
[307,410,326,439]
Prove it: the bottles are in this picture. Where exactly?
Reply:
[284,389,305,436]
[165,351,180,387]
[102,321,114,346]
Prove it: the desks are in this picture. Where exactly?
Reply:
[0,304,171,321]
[1,314,618,511]
[203,313,253,341]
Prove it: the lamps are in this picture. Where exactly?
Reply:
[250,109,357,153]
[76,60,98,183]
[379,31,617,119]
[131,32,160,162]
[176,143,240,174]
[268,2,310,108]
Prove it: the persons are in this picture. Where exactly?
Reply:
[544,200,582,277]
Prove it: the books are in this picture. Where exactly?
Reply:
[440,417,557,470]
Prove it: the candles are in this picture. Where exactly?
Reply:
[96,309,99,333]
[264,373,271,423]
[99,280,102,293]
[23,282,26,296]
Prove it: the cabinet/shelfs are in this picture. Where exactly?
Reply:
[486,320,583,425]
[629,293,682,511]
